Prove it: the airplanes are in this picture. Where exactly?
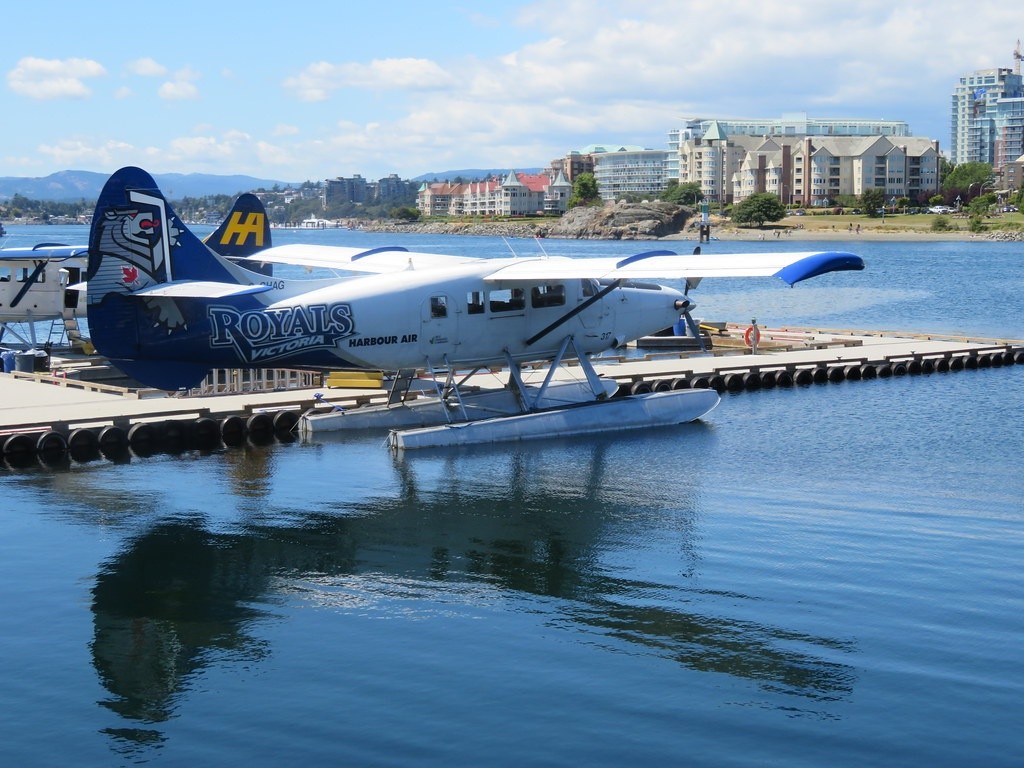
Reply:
[87,166,866,451]
[0,191,275,354]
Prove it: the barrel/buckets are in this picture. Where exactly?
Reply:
[34,356,49,371]
[686,320,700,336]
[15,354,35,373]
[3,351,16,372]
[673,319,685,336]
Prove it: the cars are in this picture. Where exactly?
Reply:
[1003,204,1021,212]
[907,205,959,215]
[852,208,862,216]
[786,208,808,217]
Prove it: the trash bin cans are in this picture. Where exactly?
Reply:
[14,353,35,373]
[34,355,48,372]
[673,319,685,336]
[684,319,701,337]
[2,351,17,373]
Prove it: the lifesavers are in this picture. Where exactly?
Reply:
[3,433,35,452]
[192,417,219,435]
[160,418,185,437]
[98,425,125,444]
[68,428,97,448]
[128,422,156,442]
[35,430,68,450]
[248,413,273,432]
[609,350,1024,398]
[743,326,760,347]
[273,411,298,429]
[219,414,245,435]
